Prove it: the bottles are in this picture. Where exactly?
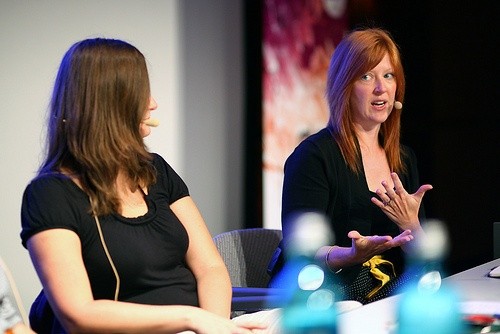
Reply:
[276,212,339,334]
[0,297,26,334]
[397,221,464,334]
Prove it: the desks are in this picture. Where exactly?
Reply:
[290,257,500,334]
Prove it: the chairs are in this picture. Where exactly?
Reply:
[211,229,293,296]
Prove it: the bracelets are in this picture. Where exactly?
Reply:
[326,245,343,275]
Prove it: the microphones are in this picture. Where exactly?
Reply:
[394,99,402,109]
[145,118,159,127]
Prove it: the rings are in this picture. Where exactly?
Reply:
[383,198,390,206]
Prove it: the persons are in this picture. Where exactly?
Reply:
[20,36,268,334]
[268,28,446,304]
